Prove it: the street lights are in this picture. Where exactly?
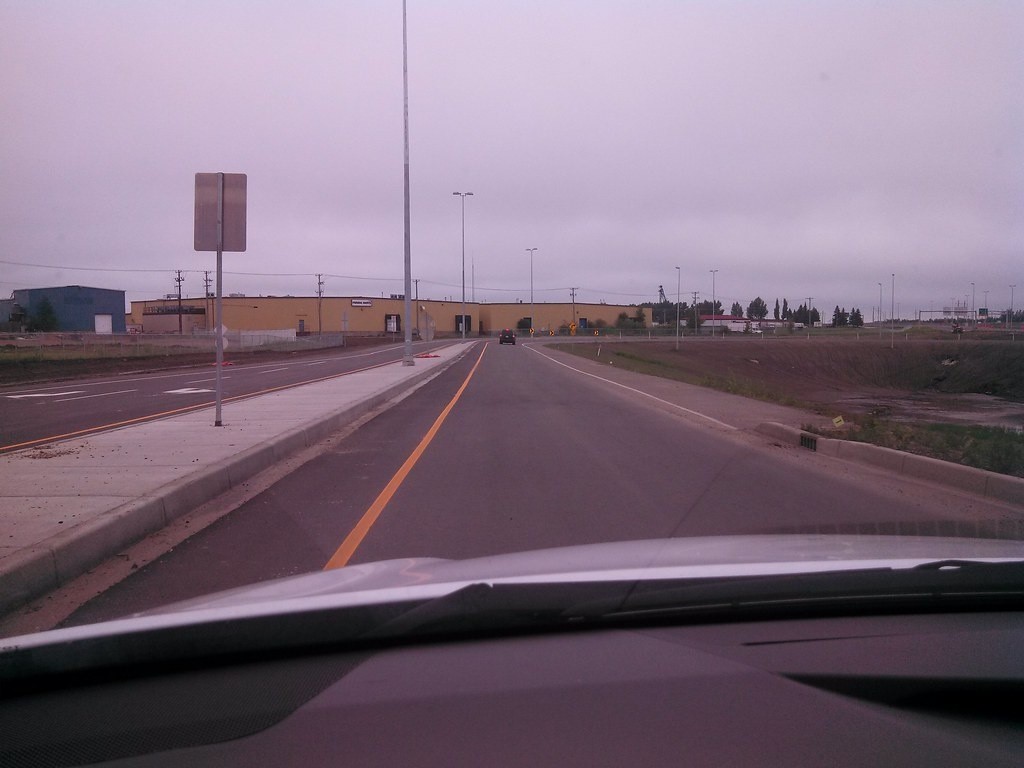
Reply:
[676,267,680,350]
[950,298,955,311]
[526,248,538,338]
[710,270,718,337]
[971,283,975,326]
[453,192,474,340]
[965,295,969,307]
[1009,285,1016,326]
[983,291,989,326]
[878,283,882,336]
[891,274,895,349]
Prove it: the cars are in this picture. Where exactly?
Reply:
[500,329,516,345]
